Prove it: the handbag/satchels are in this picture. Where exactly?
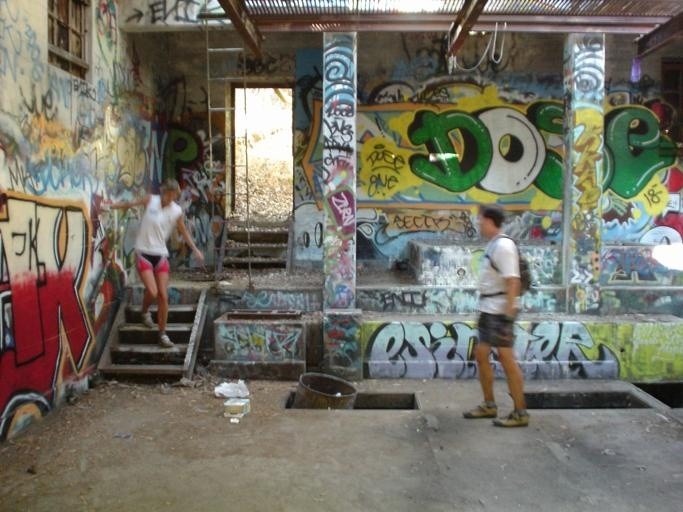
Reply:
[518,257,531,296]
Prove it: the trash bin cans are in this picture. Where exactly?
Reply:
[290,371,358,409]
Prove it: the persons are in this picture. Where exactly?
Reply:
[460,203,535,429]
[96,178,204,349]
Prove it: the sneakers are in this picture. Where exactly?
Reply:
[141,312,152,326]
[464,401,496,418]
[494,409,528,427]
[158,330,173,347]
